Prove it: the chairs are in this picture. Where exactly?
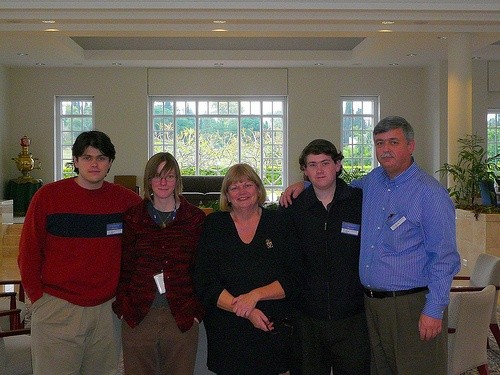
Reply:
[448,285,495,375]
[450,253,500,349]
[0,280,33,375]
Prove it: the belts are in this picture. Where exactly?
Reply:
[363,285,430,299]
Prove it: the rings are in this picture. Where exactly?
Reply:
[281,192,284,195]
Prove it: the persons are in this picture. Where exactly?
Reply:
[279,116,461,375]
[192,164,308,375]
[17,131,143,375]
[278,138,371,375]
[112,153,206,375]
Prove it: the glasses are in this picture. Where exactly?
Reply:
[151,172,176,182]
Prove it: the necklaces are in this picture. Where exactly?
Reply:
[156,209,173,229]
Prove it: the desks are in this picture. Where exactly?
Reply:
[0,200,25,224]
